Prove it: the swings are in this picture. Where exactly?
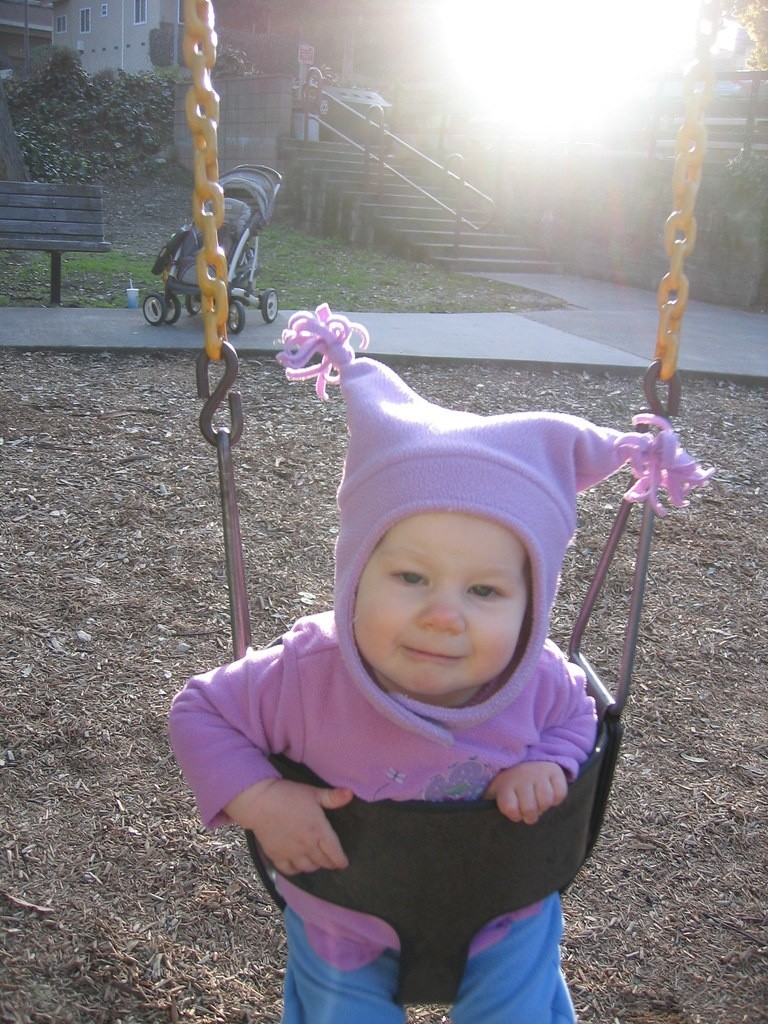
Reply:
[174,1,740,1007]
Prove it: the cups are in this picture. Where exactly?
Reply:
[126,289,139,309]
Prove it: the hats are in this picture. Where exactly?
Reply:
[276,302,718,745]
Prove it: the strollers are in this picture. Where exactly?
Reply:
[142,161,284,334]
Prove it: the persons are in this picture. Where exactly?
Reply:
[168,303,716,1024]
